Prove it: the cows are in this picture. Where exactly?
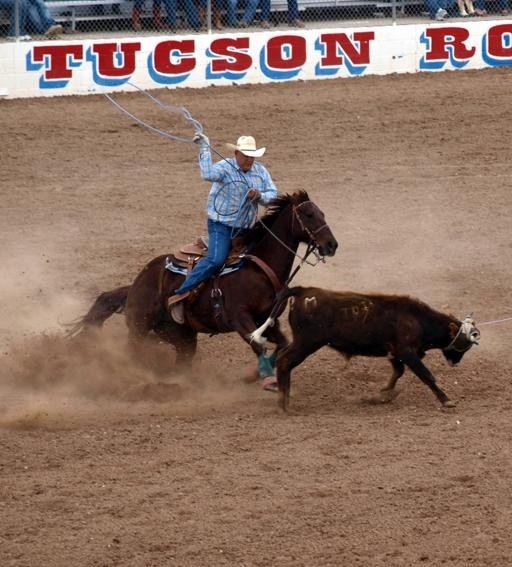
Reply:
[249,285,481,413]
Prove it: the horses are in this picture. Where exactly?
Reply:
[59,187,339,393]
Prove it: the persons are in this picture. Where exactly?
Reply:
[169,132,278,325]
[424,0,512,22]
[0,0,31,41]
[24,0,63,35]
[131,0,305,32]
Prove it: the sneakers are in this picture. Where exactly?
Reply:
[432,10,509,21]
[130,7,305,33]
[8,25,81,41]
[171,301,185,324]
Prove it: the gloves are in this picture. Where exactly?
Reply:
[192,132,209,146]
[247,189,260,200]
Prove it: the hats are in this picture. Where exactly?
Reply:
[226,135,266,158]
